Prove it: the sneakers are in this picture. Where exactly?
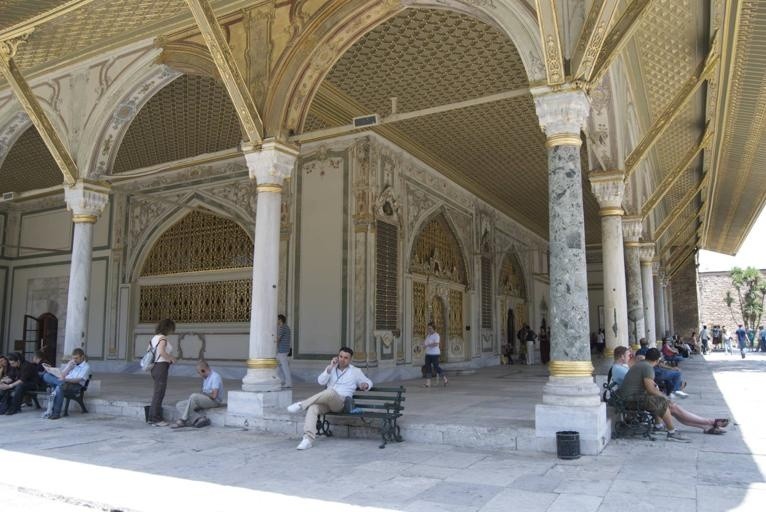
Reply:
[666,431,691,443]
[297,439,312,450]
[653,426,668,434]
[148,418,210,427]
[675,391,688,398]
[287,402,301,412]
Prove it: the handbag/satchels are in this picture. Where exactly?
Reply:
[344,396,355,414]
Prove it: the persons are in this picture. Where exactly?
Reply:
[169,362,224,428]
[286,347,373,450]
[517,323,605,365]
[276,314,294,389]
[1,337,92,420]
[423,322,448,388]
[140,319,176,426]
[611,325,766,444]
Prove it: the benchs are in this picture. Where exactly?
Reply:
[603,376,672,441]
[7,373,89,416]
[315,385,406,448]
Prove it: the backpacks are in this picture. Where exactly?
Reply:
[140,339,167,371]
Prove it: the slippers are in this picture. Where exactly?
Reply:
[713,418,728,427]
[704,426,726,434]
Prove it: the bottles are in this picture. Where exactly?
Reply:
[344,396,352,414]
